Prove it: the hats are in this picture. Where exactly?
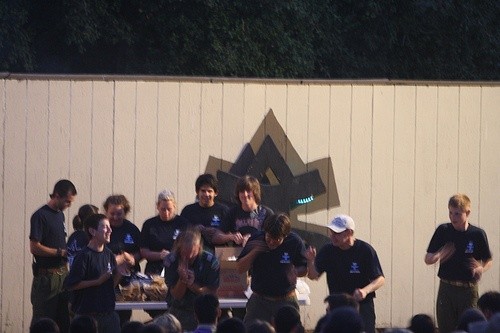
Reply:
[159,191,174,201]
[323,215,354,233]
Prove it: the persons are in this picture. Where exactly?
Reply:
[29,173,500,333]
[304,215,385,327]
[425,193,493,333]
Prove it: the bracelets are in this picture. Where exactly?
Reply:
[198,286,204,295]
[56,248,62,257]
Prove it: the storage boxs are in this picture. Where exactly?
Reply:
[216,247,250,292]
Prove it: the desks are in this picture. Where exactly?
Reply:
[116,280,309,310]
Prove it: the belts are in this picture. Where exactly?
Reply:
[36,266,68,274]
[76,310,114,318]
[253,290,295,301]
[167,302,196,311]
[440,279,476,287]
